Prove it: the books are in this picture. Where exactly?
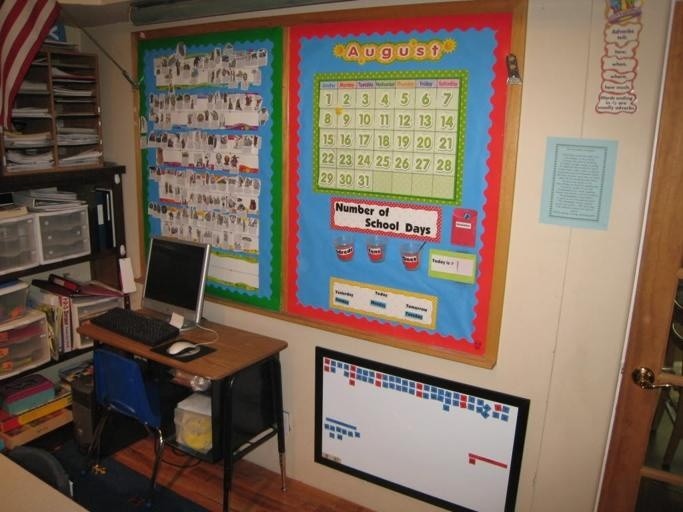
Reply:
[0,186,87,219]
[3,55,103,173]
[0,273,127,450]
[177,391,212,416]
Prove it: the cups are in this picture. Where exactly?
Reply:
[334,232,422,271]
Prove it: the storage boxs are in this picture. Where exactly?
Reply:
[0,204,125,375]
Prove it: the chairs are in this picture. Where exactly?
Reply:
[77,349,190,508]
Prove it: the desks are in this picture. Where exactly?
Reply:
[71,304,289,508]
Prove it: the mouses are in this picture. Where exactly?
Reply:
[166,341,197,356]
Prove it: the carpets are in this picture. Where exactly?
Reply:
[22,432,212,511]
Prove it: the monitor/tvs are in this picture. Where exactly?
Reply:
[141,236,212,333]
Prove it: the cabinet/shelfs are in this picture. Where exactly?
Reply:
[0,166,133,449]
[0,44,107,178]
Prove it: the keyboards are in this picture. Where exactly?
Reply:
[89,305,181,347]
